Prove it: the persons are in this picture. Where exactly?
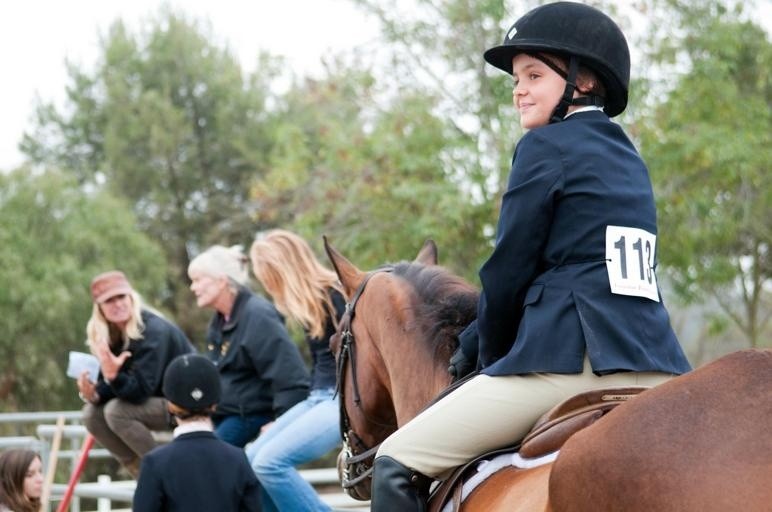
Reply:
[75,268,200,478]
[0,446,44,512]
[238,226,351,511]
[130,350,279,512]
[366,0,695,511]
[180,240,313,449]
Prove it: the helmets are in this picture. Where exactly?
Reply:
[483,0,631,118]
[160,352,222,411]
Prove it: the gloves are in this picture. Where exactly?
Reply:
[447,345,476,382]
[476,350,499,376]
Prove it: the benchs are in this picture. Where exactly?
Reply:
[0,411,379,512]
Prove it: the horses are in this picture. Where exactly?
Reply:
[322,233,772,512]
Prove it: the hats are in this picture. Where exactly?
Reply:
[90,270,131,305]
